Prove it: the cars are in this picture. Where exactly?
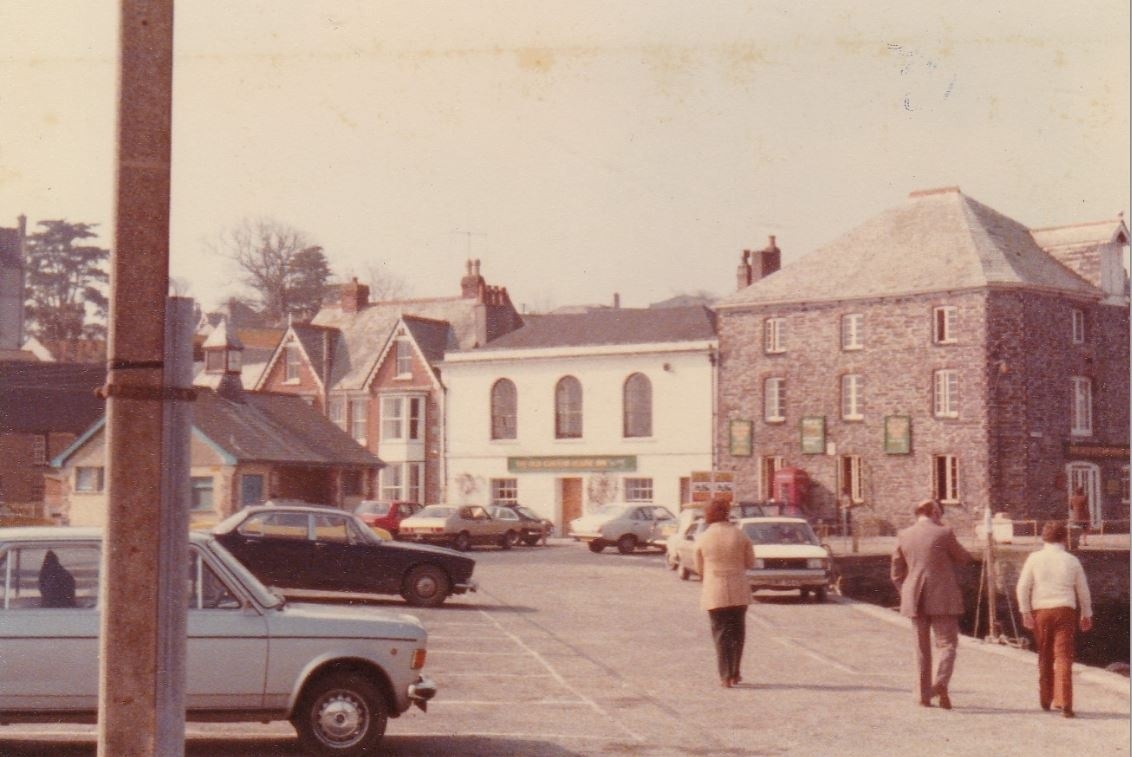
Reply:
[567,503,676,555]
[473,505,553,547]
[353,500,425,540]
[0,529,436,757]
[665,509,705,570]
[733,518,831,602]
[674,522,702,580]
[209,505,477,608]
[400,504,522,552]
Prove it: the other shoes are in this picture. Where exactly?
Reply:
[1060,709,1074,717]
[721,680,731,688]
[932,683,952,709]
[733,676,741,684]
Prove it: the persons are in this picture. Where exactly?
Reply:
[929,490,945,526]
[692,497,756,689]
[889,498,970,710]
[839,487,854,537]
[1015,518,1094,717]
[1070,486,1091,546]
